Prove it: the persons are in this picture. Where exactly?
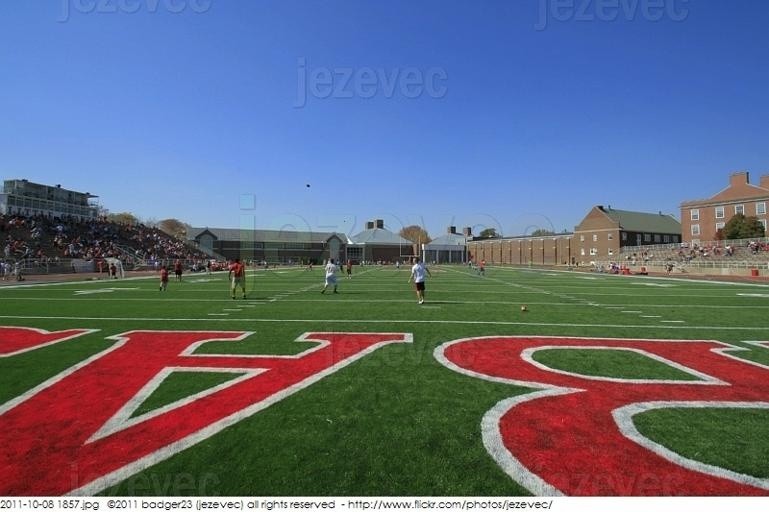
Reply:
[477,259,485,276]
[248,258,407,271]
[0,214,234,292]
[320,257,337,294]
[589,239,769,274]
[407,257,431,304]
[346,257,351,281]
[229,258,247,299]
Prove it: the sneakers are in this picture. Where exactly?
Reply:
[418,299,424,304]
[159,287,166,291]
[231,296,247,299]
[320,290,338,294]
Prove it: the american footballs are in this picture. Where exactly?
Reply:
[520,305,527,312]
[307,184,310,188]
